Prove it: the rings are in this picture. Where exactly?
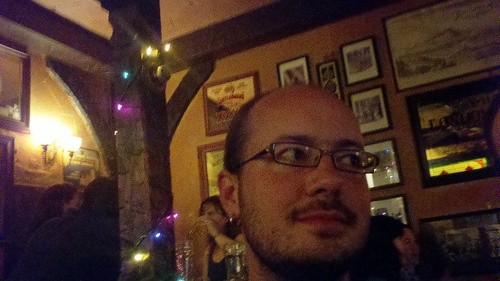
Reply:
[204,219,208,222]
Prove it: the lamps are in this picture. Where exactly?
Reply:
[141,42,171,95]
[42,135,83,169]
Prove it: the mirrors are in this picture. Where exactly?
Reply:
[0,44,32,134]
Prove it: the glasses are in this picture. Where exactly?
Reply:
[233,141,379,174]
[198,210,227,218]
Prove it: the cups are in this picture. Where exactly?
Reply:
[188,218,207,242]
[223,241,249,281]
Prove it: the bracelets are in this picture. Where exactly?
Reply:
[213,231,221,239]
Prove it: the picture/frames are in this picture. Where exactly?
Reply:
[419,207,500,281]
[317,58,345,102]
[0,134,15,184]
[339,35,382,86]
[197,140,226,202]
[370,193,411,225]
[276,54,312,89]
[62,147,101,193]
[362,137,404,191]
[381,0,500,92]
[348,84,392,136]
[203,71,261,136]
[405,74,500,188]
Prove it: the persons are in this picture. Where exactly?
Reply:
[218,83,380,281]
[12,175,247,281]
[358,214,427,281]
